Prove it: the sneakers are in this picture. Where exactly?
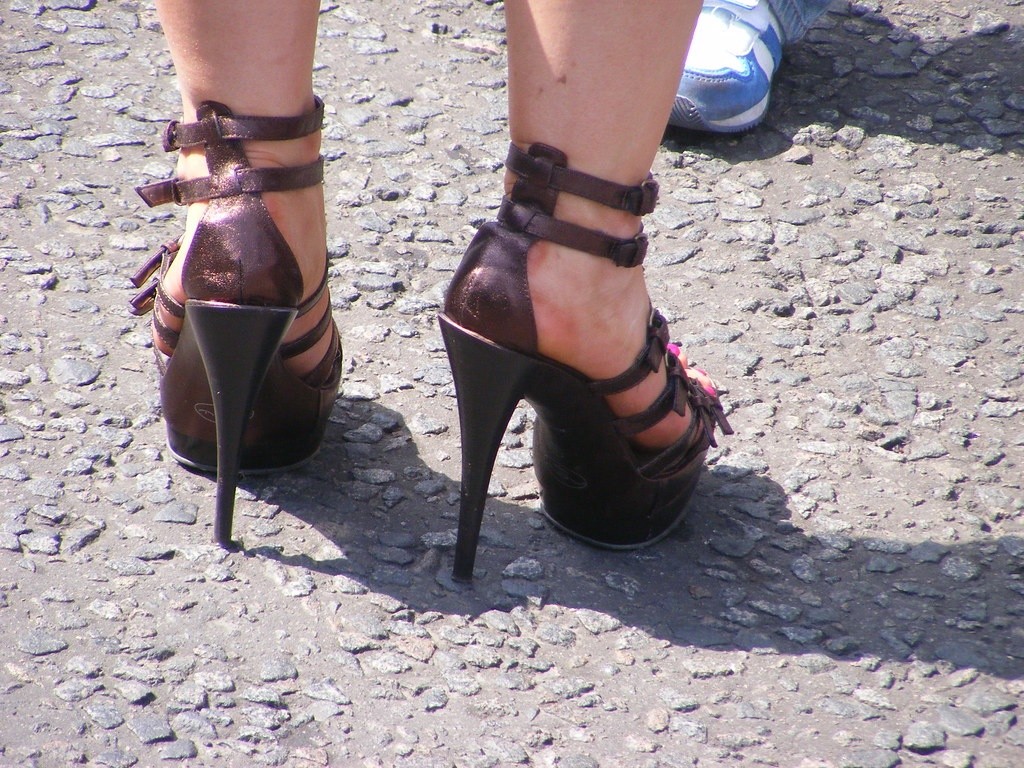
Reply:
[667,0,785,134]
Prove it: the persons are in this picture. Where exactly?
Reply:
[128,0,735,584]
[667,0,834,133]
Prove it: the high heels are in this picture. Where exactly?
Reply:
[128,88,344,544]
[436,142,734,583]
